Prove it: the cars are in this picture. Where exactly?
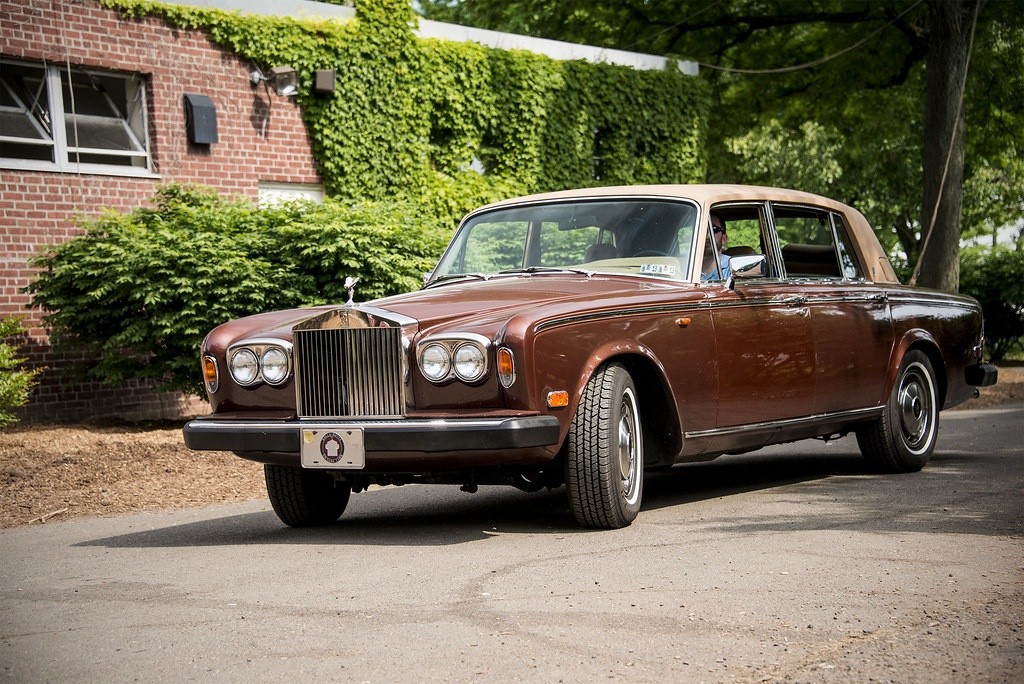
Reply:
[181,183,1000,534]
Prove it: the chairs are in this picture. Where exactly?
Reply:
[723,246,758,257]
[583,242,621,264]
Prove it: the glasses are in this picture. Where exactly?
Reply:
[712,225,724,235]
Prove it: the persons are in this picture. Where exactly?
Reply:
[685,214,732,281]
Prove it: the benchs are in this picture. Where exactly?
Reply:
[780,243,842,277]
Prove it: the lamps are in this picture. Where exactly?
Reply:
[250,64,301,97]
